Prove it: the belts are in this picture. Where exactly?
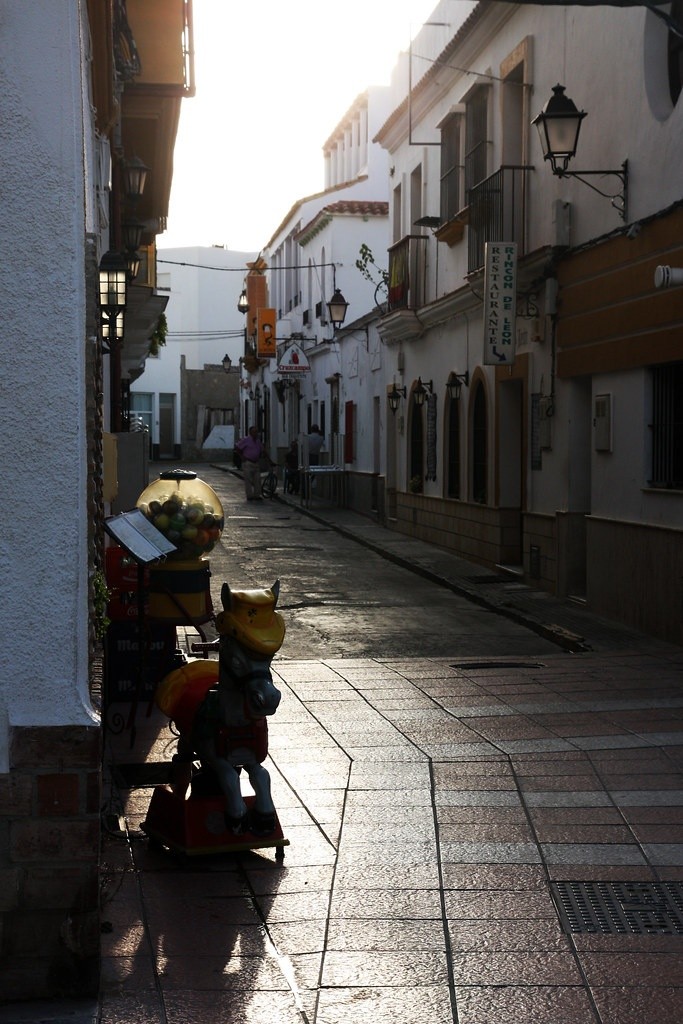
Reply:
[246,458,257,463]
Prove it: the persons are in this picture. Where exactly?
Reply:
[284,424,322,498]
[235,426,265,501]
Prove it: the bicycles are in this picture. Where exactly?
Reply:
[262,456,283,499]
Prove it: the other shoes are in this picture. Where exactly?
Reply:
[247,496,256,500]
[255,497,264,500]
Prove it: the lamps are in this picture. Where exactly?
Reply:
[222,354,242,378]
[122,148,152,204]
[412,377,433,407]
[98,245,129,354]
[388,383,406,416]
[446,370,469,401]
[530,82,629,219]
[121,208,147,283]
[326,288,369,351]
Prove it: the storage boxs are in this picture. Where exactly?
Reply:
[105,618,187,703]
[106,546,150,618]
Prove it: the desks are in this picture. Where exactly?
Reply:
[299,432,349,510]
